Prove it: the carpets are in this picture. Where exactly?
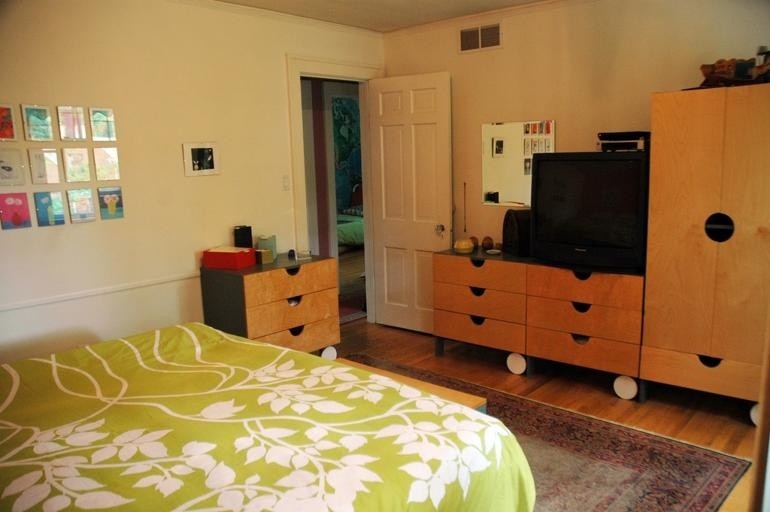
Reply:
[339,353,752,511]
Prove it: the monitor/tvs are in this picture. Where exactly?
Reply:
[529,152,649,276]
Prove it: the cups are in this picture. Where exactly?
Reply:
[234,224,254,248]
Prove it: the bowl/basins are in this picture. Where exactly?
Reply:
[454,238,475,254]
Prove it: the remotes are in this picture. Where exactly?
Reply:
[598,130,650,140]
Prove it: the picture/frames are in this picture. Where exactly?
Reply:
[181,142,220,177]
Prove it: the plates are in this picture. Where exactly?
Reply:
[485,250,501,255]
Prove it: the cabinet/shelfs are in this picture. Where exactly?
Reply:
[639,81,770,428]
[199,250,341,352]
[432,244,644,401]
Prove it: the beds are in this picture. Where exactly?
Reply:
[0,321,538,512]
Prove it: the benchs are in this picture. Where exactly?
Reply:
[336,357,487,413]
